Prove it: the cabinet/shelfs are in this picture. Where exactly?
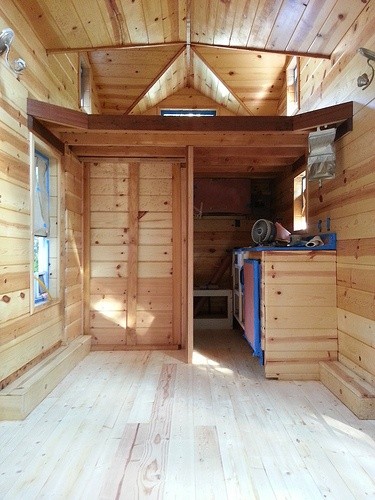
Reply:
[228,247,339,382]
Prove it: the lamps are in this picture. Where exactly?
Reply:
[355,46,375,91]
[1,27,26,73]
